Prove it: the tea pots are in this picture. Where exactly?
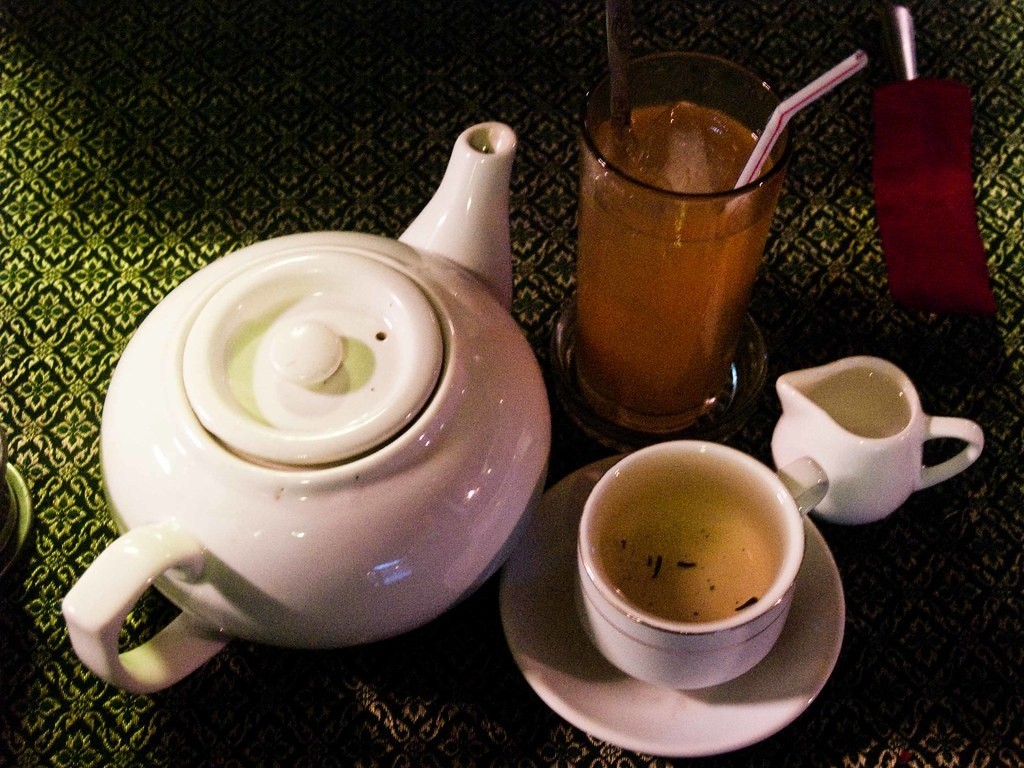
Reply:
[62,122,550,694]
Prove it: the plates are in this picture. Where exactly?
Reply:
[500,454,845,757]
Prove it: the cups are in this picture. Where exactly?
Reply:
[771,354,983,526]
[575,54,791,433]
[577,440,830,691]
